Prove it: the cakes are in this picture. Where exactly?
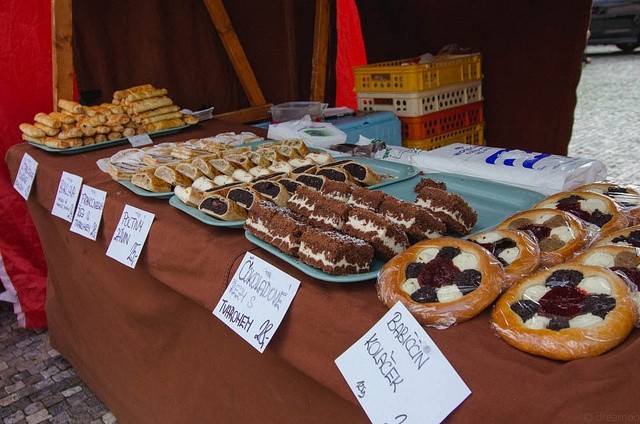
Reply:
[469,228,539,284]
[376,237,503,327]
[593,223,639,249]
[574,245,639,327]
[410,179,473,238]
[174,162,379,221]
[129,139,334,193]
[244,176,409,275]
[498,206,582,268]
[490,262,636,362]
[579,182,639,218]
[108,131,260,181]
[535,191,627,237]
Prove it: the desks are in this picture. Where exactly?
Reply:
[5,114,640,424]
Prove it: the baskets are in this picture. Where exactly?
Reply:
[402,121,487,151]
[352,52,482,94]
[356,80,484,118]
[397,100,486,141]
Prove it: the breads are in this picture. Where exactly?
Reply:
[18,83,198,150]
